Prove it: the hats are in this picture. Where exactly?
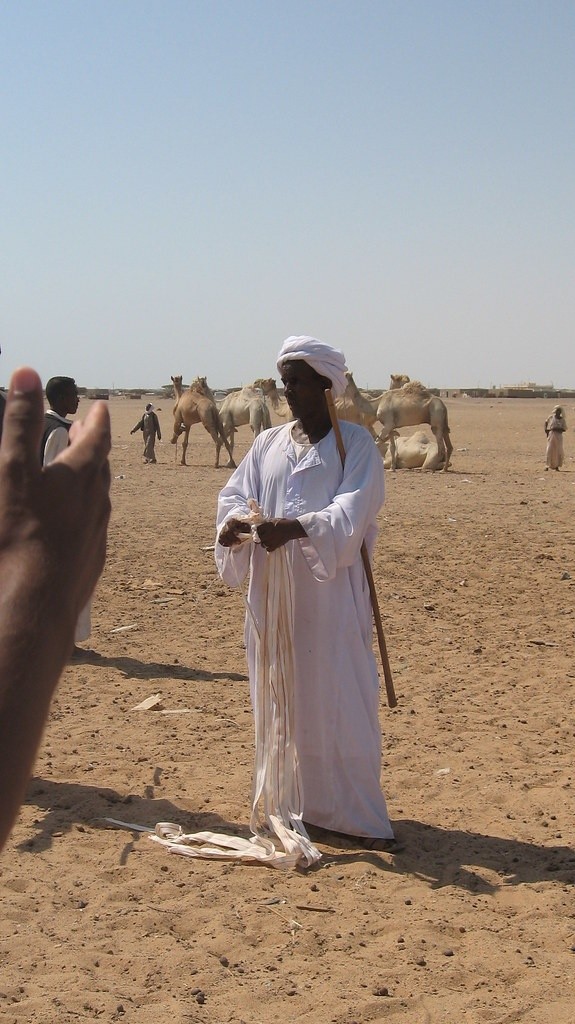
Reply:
[275,335,348,400]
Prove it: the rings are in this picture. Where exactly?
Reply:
[221,543,224,546]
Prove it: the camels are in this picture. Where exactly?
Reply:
[170,371,453,474]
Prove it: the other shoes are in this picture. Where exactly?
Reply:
[70,645,101,660]
[143,459,157,464]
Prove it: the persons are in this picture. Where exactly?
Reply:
[543,405,568,471]
[0,365,113,852]
[215,336,398,840]
[130,403,161,464]
[38,376,106,663]
